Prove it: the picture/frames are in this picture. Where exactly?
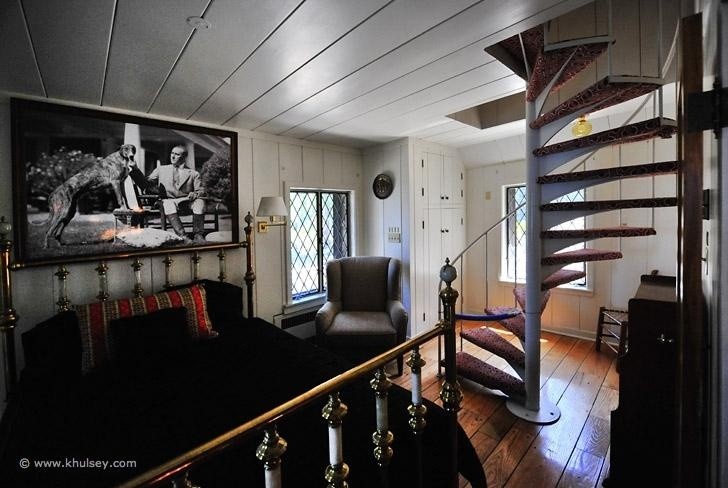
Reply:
[8,95,239,267]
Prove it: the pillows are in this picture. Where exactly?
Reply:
[74,283,220,377]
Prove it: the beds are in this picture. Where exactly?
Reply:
[0,210,464,488]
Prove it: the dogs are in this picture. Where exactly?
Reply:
[29,145,135,250]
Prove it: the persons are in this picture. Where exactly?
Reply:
[127,143,209,248]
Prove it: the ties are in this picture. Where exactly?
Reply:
[173,166,180,188]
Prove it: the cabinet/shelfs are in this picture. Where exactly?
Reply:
[360,137,467,342]
[610,273,708,487]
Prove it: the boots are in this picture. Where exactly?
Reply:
[165,212,194,246]
[193,214,206,245]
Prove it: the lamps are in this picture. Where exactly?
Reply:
[255,195,289,234]
[571,115,593,138]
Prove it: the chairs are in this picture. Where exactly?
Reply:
[315,255,408,377]
